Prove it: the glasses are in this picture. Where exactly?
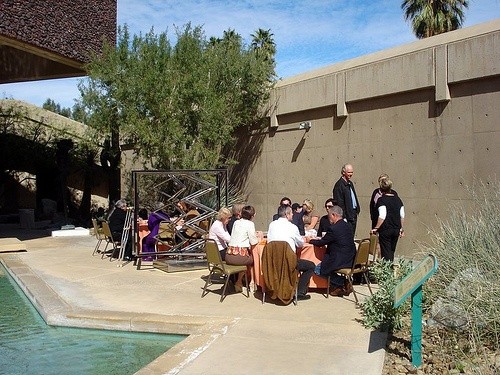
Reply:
[325,205,333,209]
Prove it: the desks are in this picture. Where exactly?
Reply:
[132,220,184,255]
[245,235,330,288]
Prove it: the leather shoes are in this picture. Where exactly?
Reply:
[297,295,311,301]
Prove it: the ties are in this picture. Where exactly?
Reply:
[349,183,357,209]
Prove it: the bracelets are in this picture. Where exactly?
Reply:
[373,227,379,230]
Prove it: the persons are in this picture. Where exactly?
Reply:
[292,203,306,236]
[226,206,258,292]
[301,199,319,230]
[209,207,238,284]
[369,177,404,270]
[261,204,316,301]
[333,164,360,238]
[142,202,170,261]
[304,206,357,296]
[168,199,201,259]
[317,199,333,240]
[106,200,133,260]
[273,197,290,221]
[226,204,242,236]
[370,173,398,258]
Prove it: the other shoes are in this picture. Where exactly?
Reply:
[142,257,153,261]
[124,256,132,261]
[331,287,346,296]
[343,284,353,295]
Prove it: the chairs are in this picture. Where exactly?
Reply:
[154,220,178,262]
[92,219,110,256]
[191,220,209,260]
[359,231,379,286]
[262,241,298,305]
[101,222,122,262]
[201,238,250,302]
[326,239,373,305]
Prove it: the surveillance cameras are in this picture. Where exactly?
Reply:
[299,121,311,130]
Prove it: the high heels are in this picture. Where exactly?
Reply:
[235,280,244,293]
[250,282,257,292]
